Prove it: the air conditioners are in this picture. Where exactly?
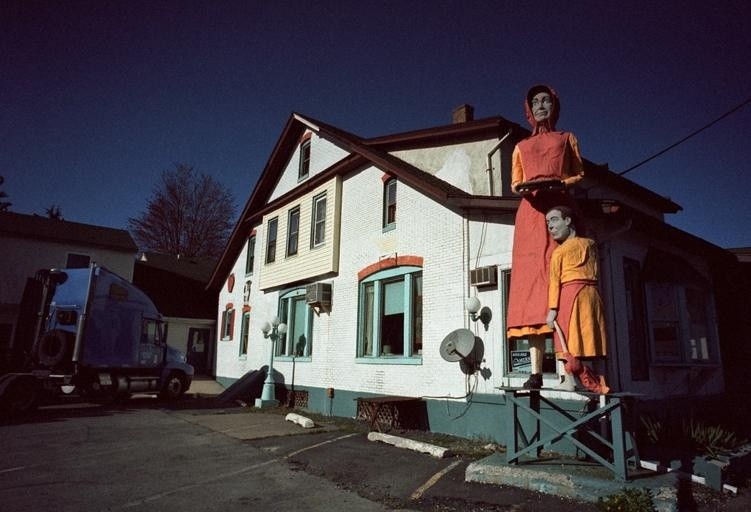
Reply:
[304,283,331,317]
[470,264,496,286]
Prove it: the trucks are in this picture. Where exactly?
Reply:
[0,259,195,421]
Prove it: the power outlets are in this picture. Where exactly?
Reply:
[466,297,491,325]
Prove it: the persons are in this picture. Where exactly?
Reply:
[505,83,585,388]
[545,206,607,361]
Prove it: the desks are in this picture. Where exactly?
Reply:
[353,395,421,435]
[494,382,645,482]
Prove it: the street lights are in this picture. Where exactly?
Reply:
[261,315,288,400]
